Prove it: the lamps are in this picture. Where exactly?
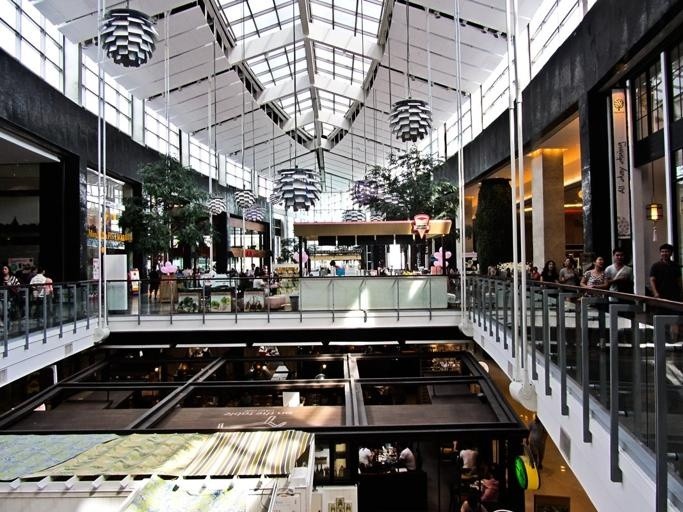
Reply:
[645,162,664,245]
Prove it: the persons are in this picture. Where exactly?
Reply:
[540,255,580,287]
[649,244,683,337]
[459,442,480,469]
[359,447,375,467]
[377,259,389,276]
[460,487,487,512]
[29,266,54,318]
[526,419,548,469]
[461,469,479,480]
[330,260,345,276]
[526,261,539,281]
[2,265,20,295]
[149,264,280,299]
[580,248,633,304]
[398,444,416,470]
[481,472,500,503]
[506,268,511,277]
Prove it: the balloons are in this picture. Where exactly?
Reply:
[433,245,451,268]
